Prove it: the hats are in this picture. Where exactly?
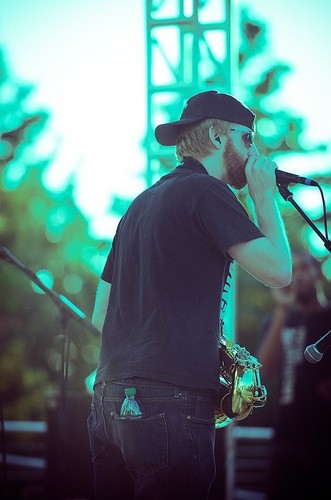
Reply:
[154,90,256,145]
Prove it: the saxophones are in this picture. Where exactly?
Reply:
[213,318,268,428]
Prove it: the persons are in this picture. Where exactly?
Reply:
[254,253,331,500]
[87,91,293,500]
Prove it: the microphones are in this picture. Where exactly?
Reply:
[304,330,330,363]
[275,168,319,186]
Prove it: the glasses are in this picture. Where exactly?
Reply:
[227,128,254,145]
